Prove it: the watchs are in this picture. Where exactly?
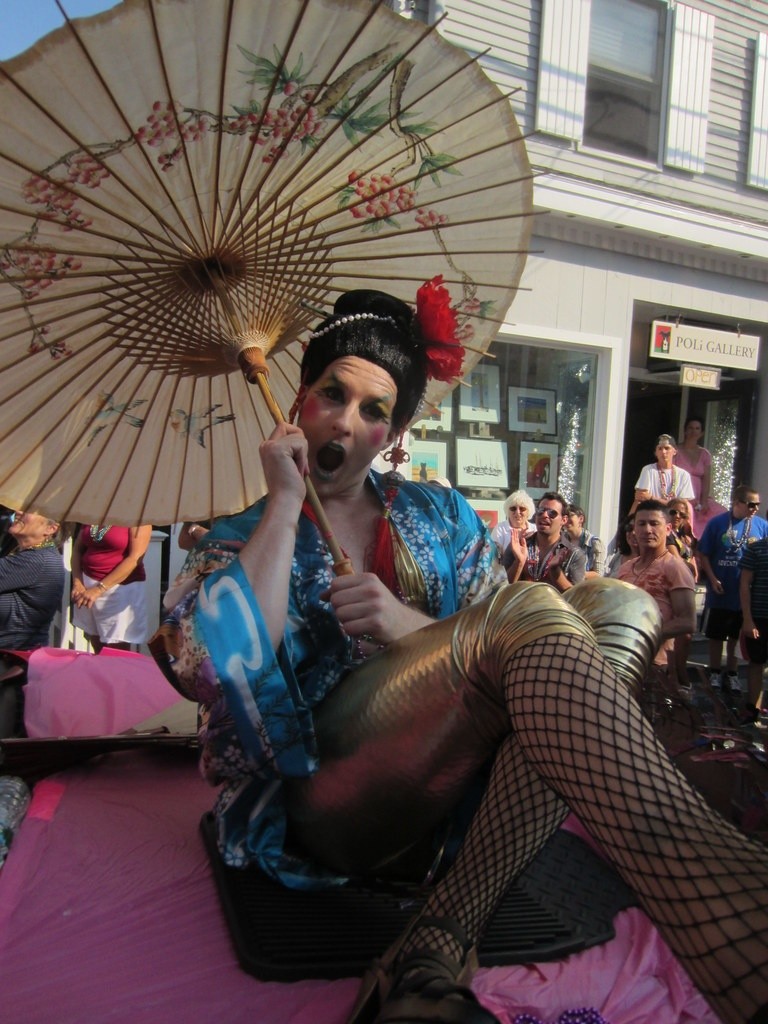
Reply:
[188,525,200,536]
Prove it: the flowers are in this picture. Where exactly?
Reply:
[415,274,466,384]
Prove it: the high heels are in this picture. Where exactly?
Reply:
[348,910,499,1024]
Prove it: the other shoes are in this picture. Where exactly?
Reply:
[678,682,696,700]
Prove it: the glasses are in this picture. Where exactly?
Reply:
[510,507,526,512]
[624,524,633,532]
[669,510,686,519]
[536,508,558,519]
[738,499,761,508]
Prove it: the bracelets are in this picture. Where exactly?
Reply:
[97,582,108,591]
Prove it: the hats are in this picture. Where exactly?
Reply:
[656,434,677,450]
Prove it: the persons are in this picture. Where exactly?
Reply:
[146,292,768,1024]
[0,510,65,650]
[428,476,451,487]
[491,490,604,593]
[179,520,213,551]
[71,525,152,652]
[605,417,768,714]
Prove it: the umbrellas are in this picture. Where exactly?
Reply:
[0,0,549,648]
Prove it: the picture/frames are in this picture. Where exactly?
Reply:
[464,496,508,529]
[506,383,558,437]
[411,438,450,484]
[409,390,454,433]
[454,435,511,490]
[517,439,560,502]
[457,362,502,425]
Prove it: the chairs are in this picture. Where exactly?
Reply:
[637,563,768,853]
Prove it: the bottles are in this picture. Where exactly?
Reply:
[0,776,33,871]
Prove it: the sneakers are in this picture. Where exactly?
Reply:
[739,701,763,728]
[709,668,722,691]
[727,671,743,697]
